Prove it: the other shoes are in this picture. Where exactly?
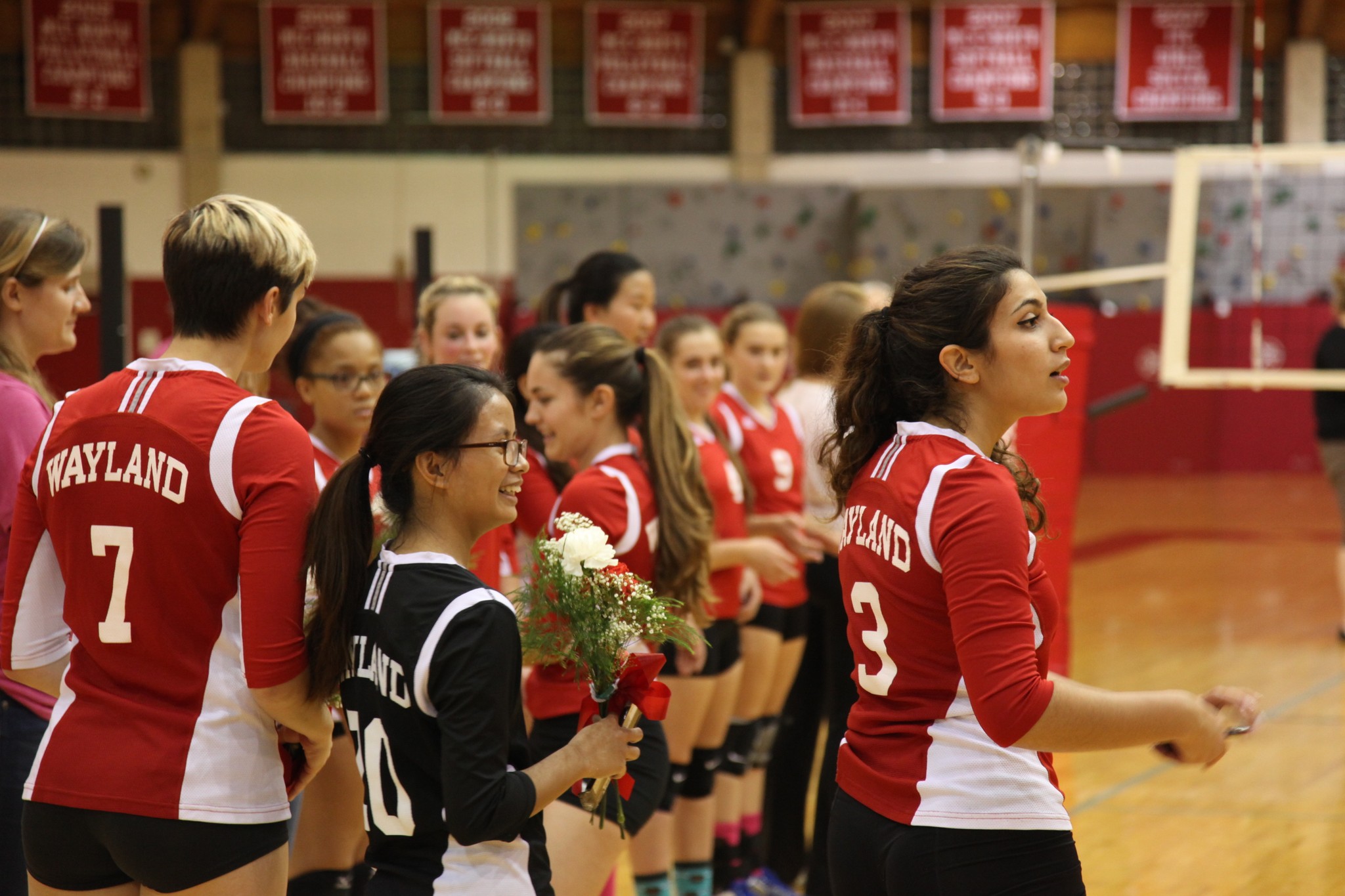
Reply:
[718,866,796,896]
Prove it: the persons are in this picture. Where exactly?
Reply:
[301,365,644,896]
[1308,259,1345,642]
[703,301,838,896]
[0,194,1263,896]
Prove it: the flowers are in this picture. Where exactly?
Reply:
[507,511,713,841]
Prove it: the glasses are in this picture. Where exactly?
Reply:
[439,439,528,467]
[302,366,387,394]
